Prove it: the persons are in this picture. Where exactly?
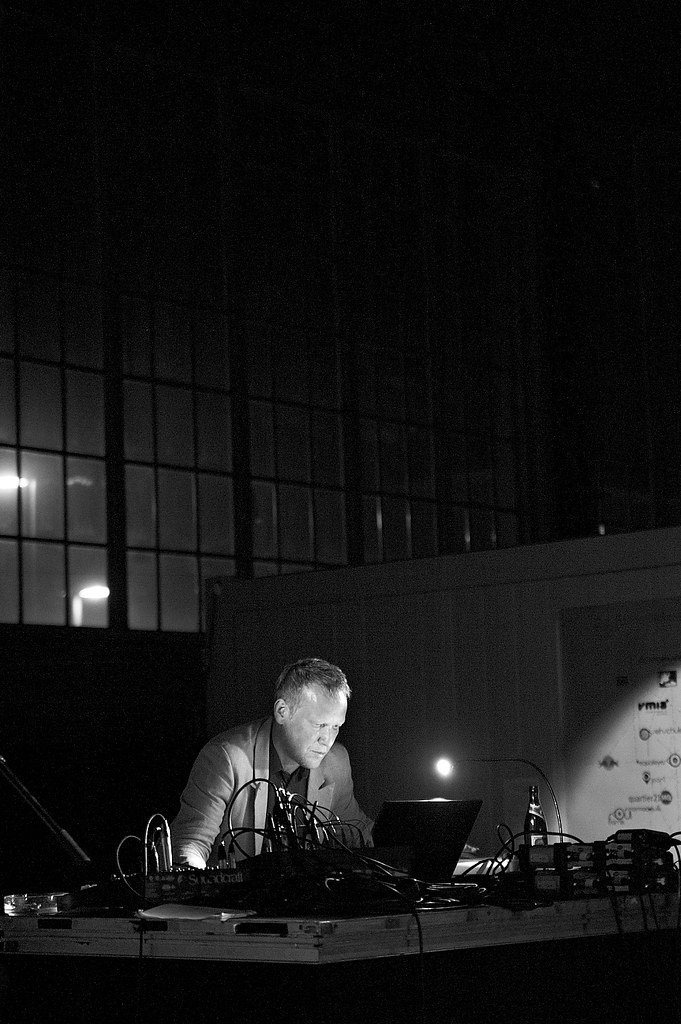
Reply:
[159,658,374,873]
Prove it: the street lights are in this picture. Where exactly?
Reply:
[71,585,109,629]
[0,473,36,592]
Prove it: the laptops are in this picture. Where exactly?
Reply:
[371,797,484,883]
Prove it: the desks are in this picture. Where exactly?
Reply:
[0,852,681,1023]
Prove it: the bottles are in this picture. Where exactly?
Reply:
[523,784,550,848]
